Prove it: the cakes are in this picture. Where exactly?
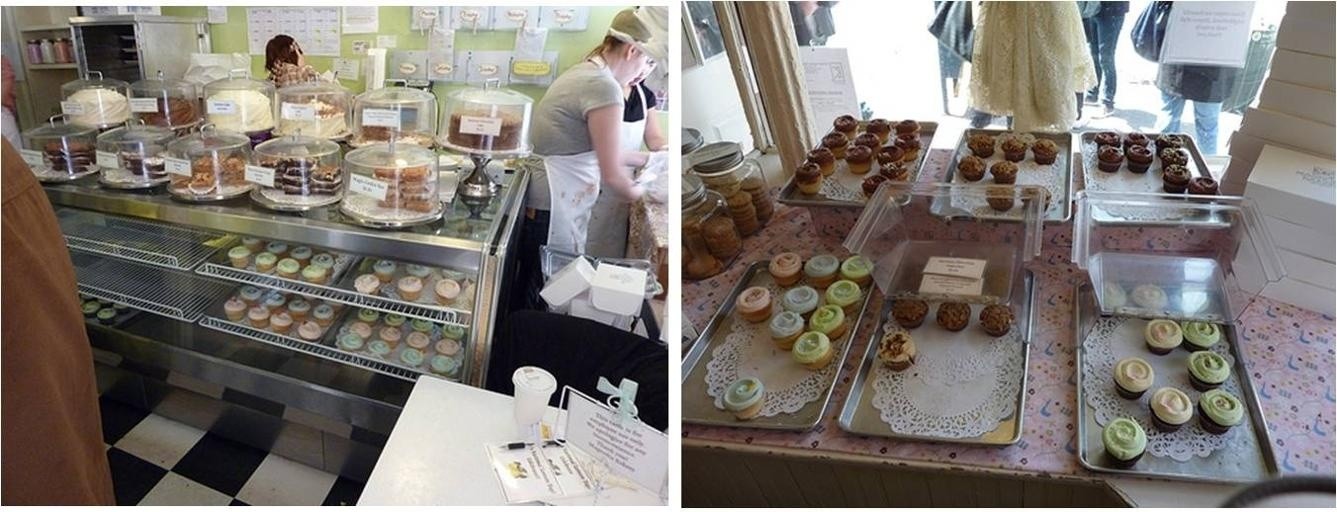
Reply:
[878,299,1013,370]
[355,258,479,303]
[1095,132,1216,193]
[721,377,765,419]
[736,252,872,371]
[796,115,921,197]
[43,88,521,211]
[957,136,1059,210]
[230,237,338,283]
[79,295,129,324]
[224,287,344,340]
[1093,281,1243,470]
[342,308,465,379]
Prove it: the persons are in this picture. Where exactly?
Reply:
[1076,1,1131,124]
[1151,56,1239,158]
[0,123,122,506]
[521,7,656,292]
[583,31,666,268]
[257,28,337,91]
[0,56,22,156]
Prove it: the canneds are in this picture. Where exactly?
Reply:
[26,38,74,63]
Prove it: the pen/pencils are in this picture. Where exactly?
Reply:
[501,441,566,450]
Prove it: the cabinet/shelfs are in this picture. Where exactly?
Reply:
[19,20,78,72]
[29,165,528,482]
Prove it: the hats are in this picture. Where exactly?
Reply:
[608,7,667,60]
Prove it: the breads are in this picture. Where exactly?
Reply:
[682,171,773,278]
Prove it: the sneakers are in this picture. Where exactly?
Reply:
[942,84,1117,130]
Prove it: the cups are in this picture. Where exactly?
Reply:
[509,363,558,428]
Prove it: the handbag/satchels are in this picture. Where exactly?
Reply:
[928,2,972,61]
[1131,1,1173,61]
